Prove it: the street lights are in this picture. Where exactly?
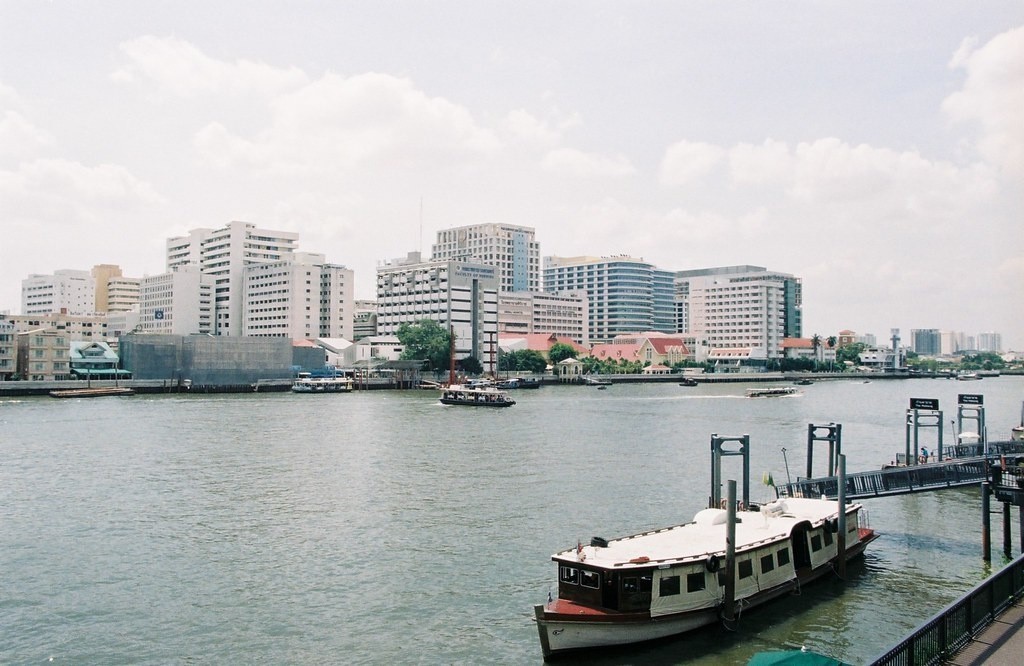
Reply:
[950,419,958,446]
[782,447,791,484]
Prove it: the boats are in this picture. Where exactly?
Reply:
[793,378,814,385]
[746,386,798,398]
[464,376,542,390]
[985,454,1024,507]
[292,369,355,394]
[439,383,517,407]
[679,377,699,387]
[530,496,881,666]
[49,367,136,398]
[879,452,916,470]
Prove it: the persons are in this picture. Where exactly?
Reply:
[921,448,928,463]
